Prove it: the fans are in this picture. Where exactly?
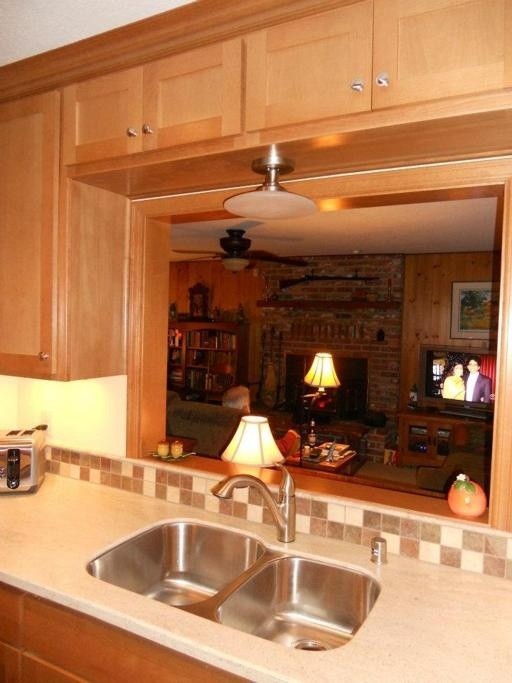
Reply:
[171,228,309,266]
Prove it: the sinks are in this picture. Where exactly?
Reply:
[213,554,380,652]
[84,520,269,607]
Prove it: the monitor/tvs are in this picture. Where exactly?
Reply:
[416,343,496,421]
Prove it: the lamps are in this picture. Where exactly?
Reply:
[222,258,249,272]
[223,156,317,220]
[220,416,285,468]
[303,352,340,394]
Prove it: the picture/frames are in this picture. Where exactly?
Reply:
[450,280,500,340]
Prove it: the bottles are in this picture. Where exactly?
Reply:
[307,417,318,447]
[157,439,170,458]
[171,439,184,459]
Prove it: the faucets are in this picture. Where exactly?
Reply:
[209,464,297,543]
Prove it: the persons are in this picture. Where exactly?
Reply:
[440,360,467,400]
[223,385,256,416]
[465,357,493,402]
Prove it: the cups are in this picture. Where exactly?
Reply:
[302,445,312,458]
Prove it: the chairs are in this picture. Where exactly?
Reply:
[167,401,244,458]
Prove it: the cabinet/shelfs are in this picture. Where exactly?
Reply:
[167,322,248,401]
[240,0,512,185]
[395,409,492,468]
[63,39,242,200]
[0,89,124,381]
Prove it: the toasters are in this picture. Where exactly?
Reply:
[0,423,52,498]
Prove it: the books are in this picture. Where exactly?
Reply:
[320,441,354,460]
[167,325,234,398]
